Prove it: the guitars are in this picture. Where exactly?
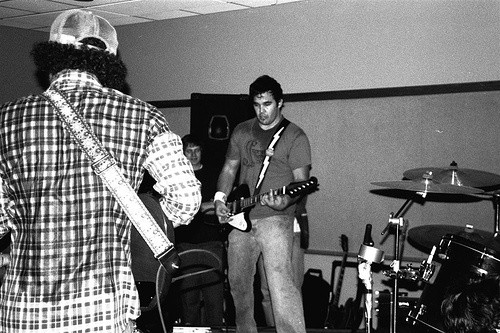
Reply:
[226,176,318,232]
[131,193,173,311]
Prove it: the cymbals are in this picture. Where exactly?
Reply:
[402,166,500,189]
[408,224,500,251]
[370,180,486,195]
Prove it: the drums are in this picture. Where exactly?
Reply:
[406,233,500,333]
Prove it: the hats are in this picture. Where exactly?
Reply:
[47,9,118,56]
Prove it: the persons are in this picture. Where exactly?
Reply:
[173,132,226,332]
[214,74,313,333]
[0,10,203,333]
